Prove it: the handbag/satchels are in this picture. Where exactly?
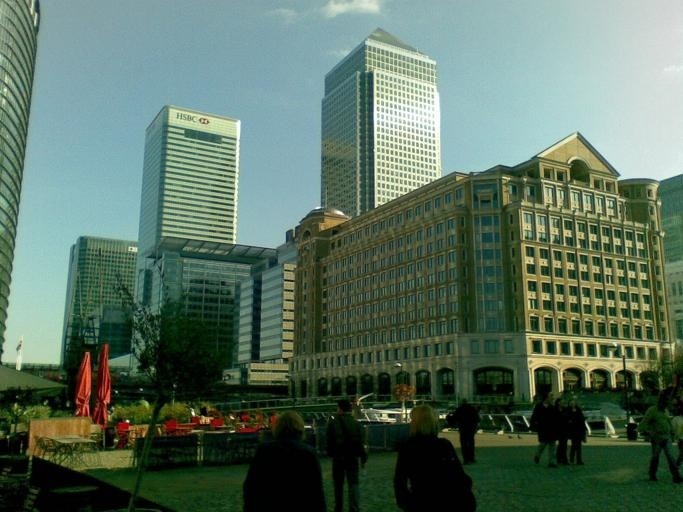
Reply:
[438,465,477,512]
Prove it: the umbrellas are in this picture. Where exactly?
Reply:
[73,350,92,417]
[92,342,112,427]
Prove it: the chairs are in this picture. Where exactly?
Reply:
[32,432,104,470]
[115,413,278,465]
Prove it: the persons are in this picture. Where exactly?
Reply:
[391,403,478,510]
[568,399,586,466]
[529,390,557,468]
[453,397,481,465]
[554,397,569,465]
[242,409,328,511]
[637,391,682,483]
[325,398,366,511]
[670,404,682,466]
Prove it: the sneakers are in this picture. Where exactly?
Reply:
[648,471,658,481]
[672,474,683,484]
[534,453,585,467]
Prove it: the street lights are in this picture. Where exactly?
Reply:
[393,361,406,422]
[607,342,629,422]
